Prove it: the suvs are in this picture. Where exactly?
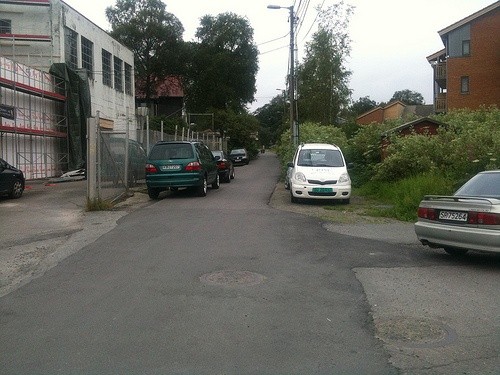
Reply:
[144,142,221,199]
[229,147,249,165]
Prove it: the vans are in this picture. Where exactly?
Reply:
[96,136,146,181]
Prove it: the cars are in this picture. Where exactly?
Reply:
[285,142,351,204]
[0,159,25,200]
[211,150,235,183]
[413,170,499,257]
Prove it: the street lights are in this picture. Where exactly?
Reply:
[267,3,297,144]
